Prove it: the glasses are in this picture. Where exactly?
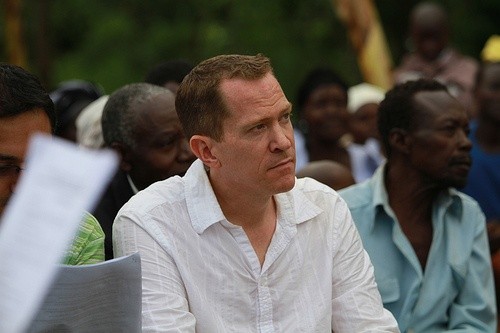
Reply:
[0,157,21,176]
